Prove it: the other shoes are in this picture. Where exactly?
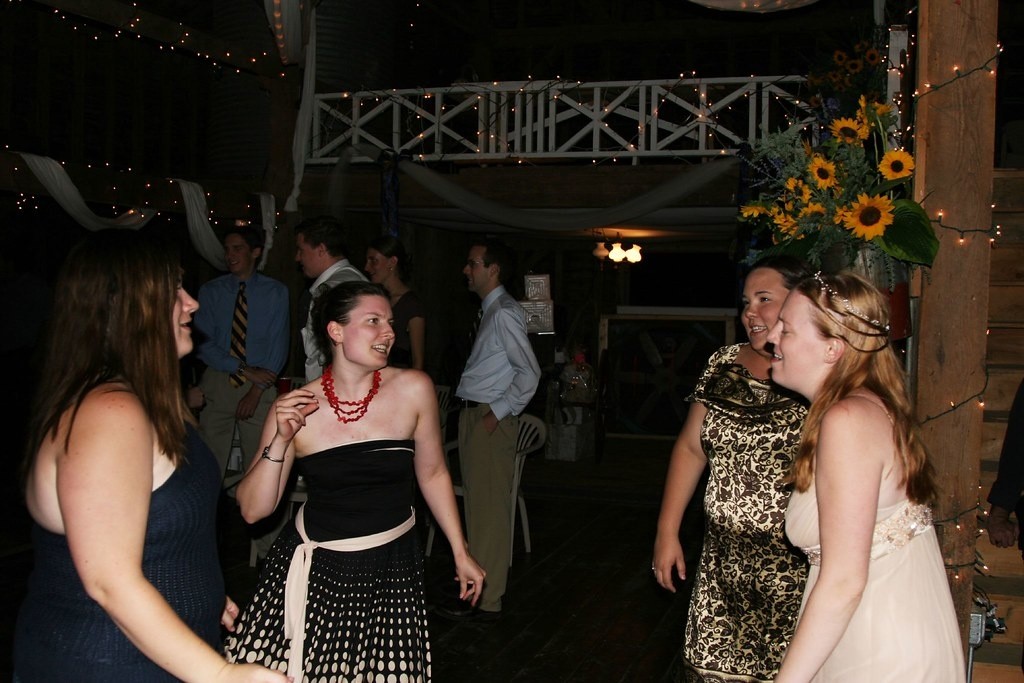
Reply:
[435,598,503,623]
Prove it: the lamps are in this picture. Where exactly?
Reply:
[592,225,643,272]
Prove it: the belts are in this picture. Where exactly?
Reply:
[457,397,480,408]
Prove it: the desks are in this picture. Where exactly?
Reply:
[596,306,738,440]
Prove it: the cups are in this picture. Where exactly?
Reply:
[277,377,291,395]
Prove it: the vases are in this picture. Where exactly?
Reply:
[825,239,912,344]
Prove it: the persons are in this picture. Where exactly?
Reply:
[363,235,427,370]
[764,271,968,683]
[434,241,542,622]
[180,225,290,560]
[292,215,368,384]
[224,281,486,674]
[13,228,293,683]
[651,254,813,683]
[985,377,1024,562]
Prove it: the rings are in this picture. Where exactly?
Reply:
[652,568,656,570]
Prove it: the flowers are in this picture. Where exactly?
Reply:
[731,23,941,280]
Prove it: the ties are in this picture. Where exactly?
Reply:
[228,283,247,387]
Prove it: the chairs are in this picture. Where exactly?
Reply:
[553,348,612,465]
[422,384,545,558]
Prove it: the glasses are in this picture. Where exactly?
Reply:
[467,260,486,267]
[365,257,389,265]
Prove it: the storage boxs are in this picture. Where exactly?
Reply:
[546,419,595,461]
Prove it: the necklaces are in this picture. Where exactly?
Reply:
[321,364,381,423]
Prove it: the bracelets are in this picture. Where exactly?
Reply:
[262,447,285,462]
[238,364,246,373]
